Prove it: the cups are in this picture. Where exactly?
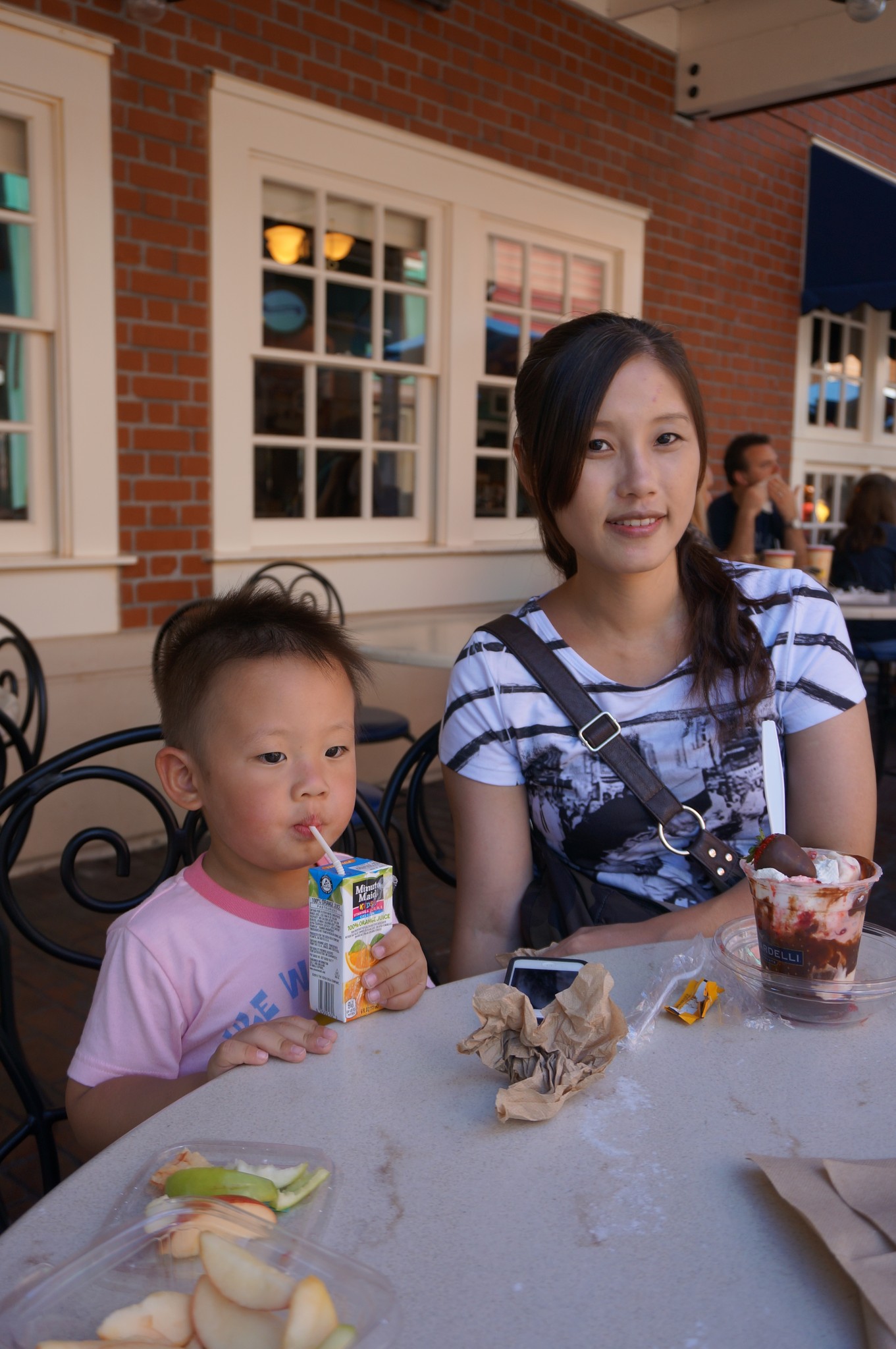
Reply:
[739,847,883,1023]
[806,544,834,589]
[764,549,797,569]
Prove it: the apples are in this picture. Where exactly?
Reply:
[50,1225,348,1346]
[132,1152,330,1265]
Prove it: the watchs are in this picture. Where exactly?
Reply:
[783,518,802,534]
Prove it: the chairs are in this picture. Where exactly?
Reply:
[0,722,414,1197]
[236,561,446,886]
[373,717,458,991]
[0,611,51,883]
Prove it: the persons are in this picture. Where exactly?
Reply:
[68,592,434,1157]
[832,472,896,660]
[687,458,716,555]
[438,312,884,980]
[254,404,533,524]
[711,431,812,575]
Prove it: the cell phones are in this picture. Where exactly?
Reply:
[502,956,588,1026]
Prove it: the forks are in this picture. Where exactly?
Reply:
[637,958,707,1036]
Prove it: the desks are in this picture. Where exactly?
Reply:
[0,935,896,1348]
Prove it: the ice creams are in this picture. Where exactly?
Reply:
[733,828,877,1015]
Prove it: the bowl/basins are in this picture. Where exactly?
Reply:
[710,913,896,1023]
